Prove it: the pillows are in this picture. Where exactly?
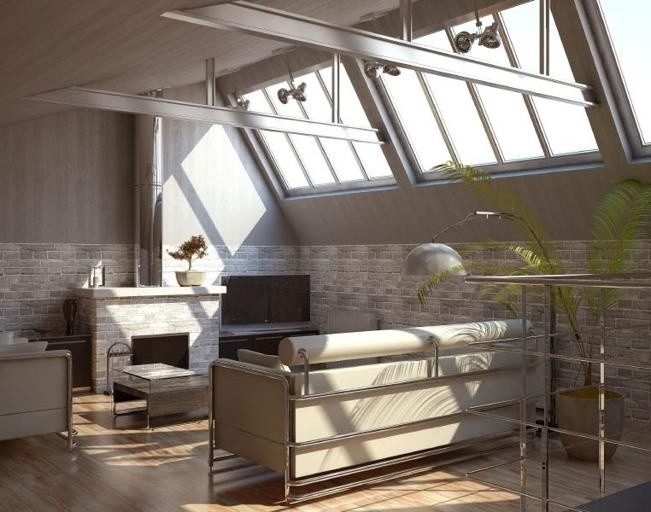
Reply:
[1,329,49,355]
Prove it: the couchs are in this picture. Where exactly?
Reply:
[206,317,541,507]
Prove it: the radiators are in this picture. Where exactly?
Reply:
[325,311,382,368]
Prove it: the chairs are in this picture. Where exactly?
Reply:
[0,329,79,456]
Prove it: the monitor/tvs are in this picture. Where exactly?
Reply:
[221,274,311,335]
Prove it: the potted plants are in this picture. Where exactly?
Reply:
[405,156,650,463]
[164,235,213,289]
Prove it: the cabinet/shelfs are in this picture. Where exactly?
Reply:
[219,323,320,369]
[28,333,94,395]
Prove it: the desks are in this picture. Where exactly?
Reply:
[110,363,210,430]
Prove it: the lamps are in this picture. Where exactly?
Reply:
[220,66,251,110]
[405,207,589,389]
[444,1,510,53]
[262,47,310,102]
[350,9,403,82]
